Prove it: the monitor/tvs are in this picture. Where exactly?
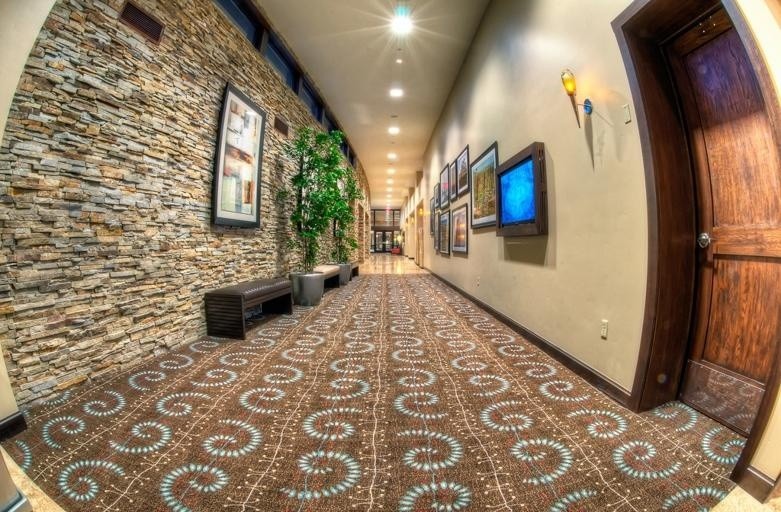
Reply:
[494,141,548,236]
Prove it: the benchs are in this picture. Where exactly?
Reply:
[205,279,293,340]
[349,260,359,281]
[313,264,341,297]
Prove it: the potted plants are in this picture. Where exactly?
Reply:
[330,167,364,285]
[278,126,345,306]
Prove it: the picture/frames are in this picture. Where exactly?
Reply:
[211,82,266,228]
[430,141,499,256]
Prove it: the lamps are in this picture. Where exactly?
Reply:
[560,68,592,128]
